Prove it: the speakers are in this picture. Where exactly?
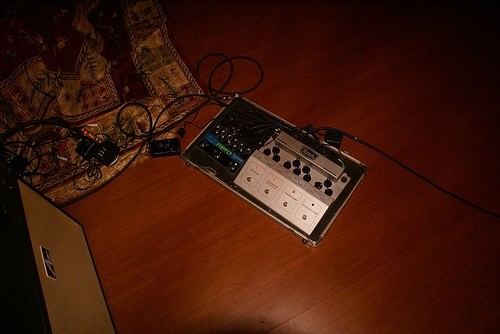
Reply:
[1,176,116,333]
